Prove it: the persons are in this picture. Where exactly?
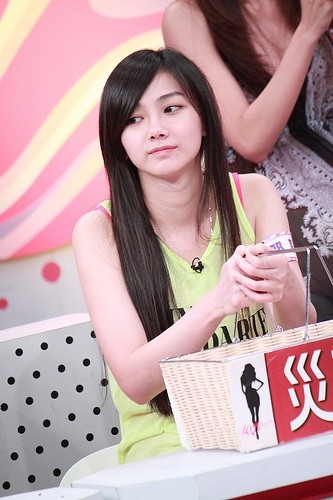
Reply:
[72,49,317,464]
[161,0,333,323]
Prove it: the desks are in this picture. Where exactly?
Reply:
[0,431,333,500]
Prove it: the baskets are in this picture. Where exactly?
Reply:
[158,245,333,452]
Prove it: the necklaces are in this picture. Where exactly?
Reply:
[153,193,214,247]
